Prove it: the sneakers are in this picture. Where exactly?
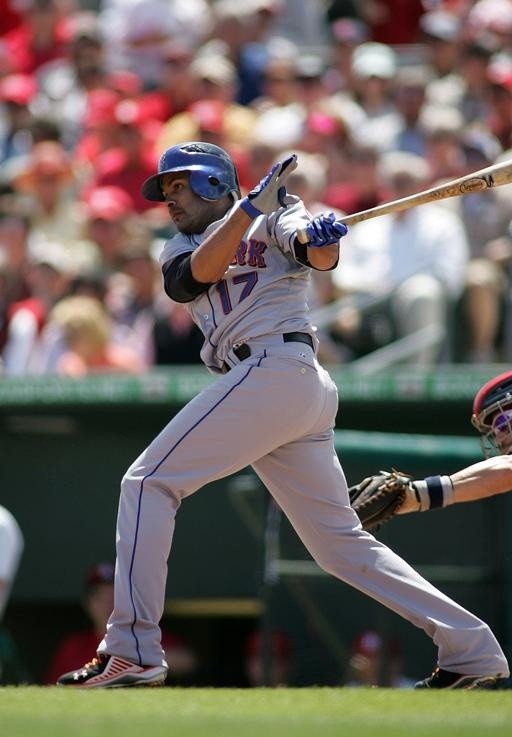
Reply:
[409,652,507,692]
[53,646,166,692]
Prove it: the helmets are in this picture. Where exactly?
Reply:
[468,369,511,459]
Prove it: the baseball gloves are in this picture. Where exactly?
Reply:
[349,471,410,529]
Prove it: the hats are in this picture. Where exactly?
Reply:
[247,626,402,664]
[0,0,511,280]
[81,563,115,584]
[140,141,241,206]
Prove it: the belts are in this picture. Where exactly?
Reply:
[217,329,317,375]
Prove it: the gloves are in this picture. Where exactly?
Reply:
[305,211,349,249]
[238,153,303,220]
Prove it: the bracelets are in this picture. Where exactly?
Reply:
[413,471,456,514]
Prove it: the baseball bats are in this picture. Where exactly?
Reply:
[296,162,512,245]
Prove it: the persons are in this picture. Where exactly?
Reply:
[56,141,512,690]
[244,630,294,687]
[1,0,512,382]
[46,561,204,688]
[0,504,28,623]
[339,627,422,687]
[344,368,512,529]
